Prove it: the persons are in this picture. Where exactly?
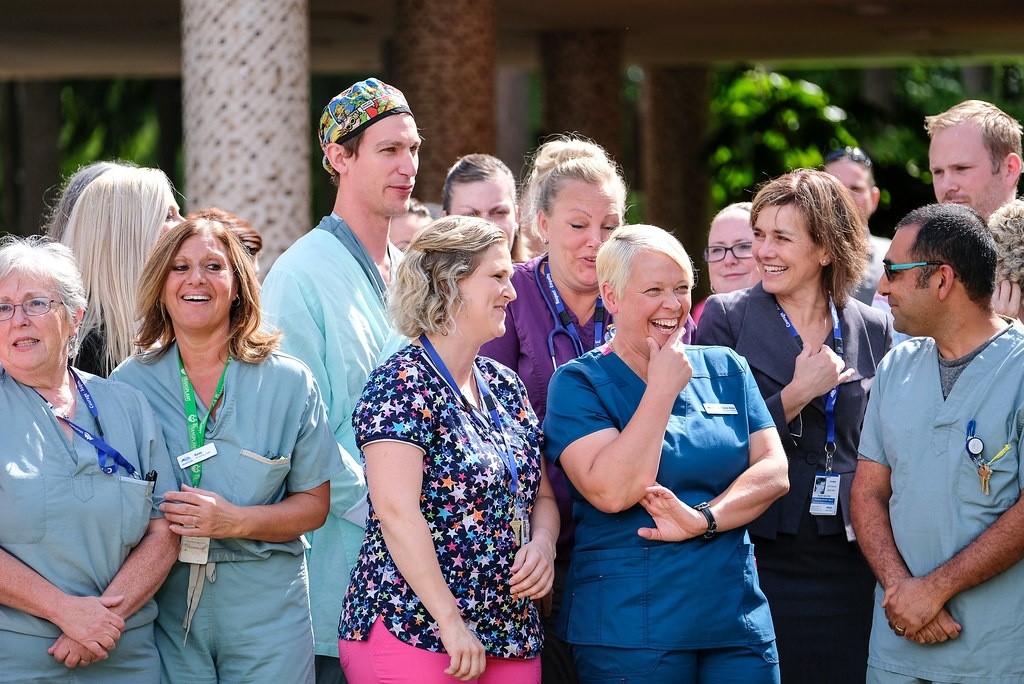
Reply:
[705,202,762,294]
[260,78,421,684]
[542,223,790,684]
[849,203,1024,684]
[0,233,181,684]
[818,146,893,307]
[337,216,559,684]
[45,161,263,379]
[106,218,345,684]
[695,168,893,684]
[389,153,523,263]
[892,99,1024,346]
[814,477,826,494]
[478,138,697,684]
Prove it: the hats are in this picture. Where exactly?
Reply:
[317,78,415,175]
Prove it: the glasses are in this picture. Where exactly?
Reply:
[703,242,753,262]
[0,297,63,321]
[882,260,957,281]
[827,147,873,168]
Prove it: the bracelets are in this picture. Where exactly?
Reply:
[692,502,717,540]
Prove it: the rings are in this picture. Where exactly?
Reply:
[895,625,905,632]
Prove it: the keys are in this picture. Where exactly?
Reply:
[978,466,993,495]
